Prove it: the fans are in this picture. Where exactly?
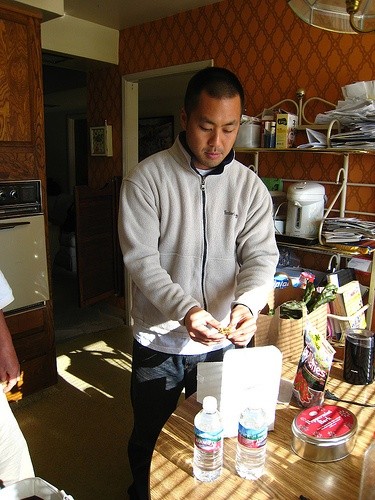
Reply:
[286,0,375,35]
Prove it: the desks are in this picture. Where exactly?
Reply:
[149,360,375,500]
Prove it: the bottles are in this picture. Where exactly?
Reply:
[193,396,223,482]
[235,401,267,480]
[358,432,375,500]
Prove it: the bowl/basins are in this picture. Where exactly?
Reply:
[290,405,359,463]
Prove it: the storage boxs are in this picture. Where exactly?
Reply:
[327,269,367,341]
[197,345,283,440]
[261,108,298,149]
[253,287,328,360]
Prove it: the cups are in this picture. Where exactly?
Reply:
[343,328,375,385]
[274,220,284,235]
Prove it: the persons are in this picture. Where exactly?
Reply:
[118,66,280,500]
[0,271,35,488]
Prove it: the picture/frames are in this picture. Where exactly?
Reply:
[139,115,174,162]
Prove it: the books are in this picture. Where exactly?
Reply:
[319,216,375,341]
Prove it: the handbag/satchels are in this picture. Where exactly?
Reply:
[252,272,327,380]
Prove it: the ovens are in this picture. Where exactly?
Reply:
[0,180,50,319]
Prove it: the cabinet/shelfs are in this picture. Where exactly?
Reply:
[0,2,58,402]
[234,150,375,347]
